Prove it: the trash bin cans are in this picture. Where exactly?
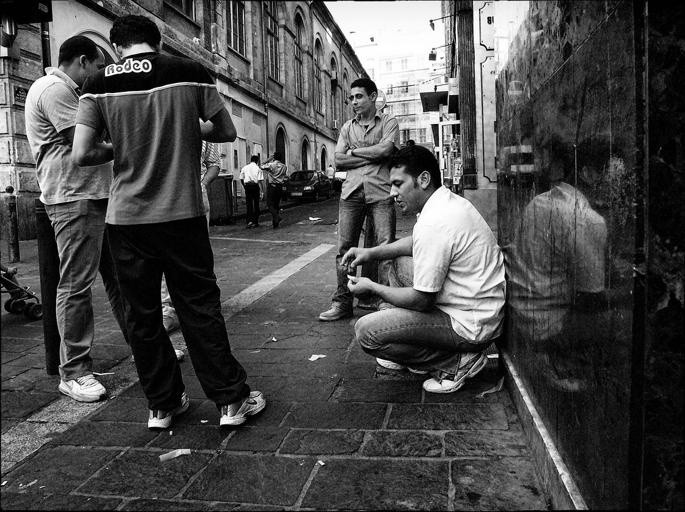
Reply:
[207,174,234,226]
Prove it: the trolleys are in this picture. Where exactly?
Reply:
[0,264,43,320]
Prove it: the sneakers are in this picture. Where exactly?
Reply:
[319,302,353,321]
[219,389,267,429]
[423,352,490,394]
[376,357,431,375]
[58,374,108,402]
[130,348,184,362]
[148,392,191,432]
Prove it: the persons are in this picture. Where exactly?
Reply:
[70,14,266,429]
[239,156,264,229]
[340,144,507,393]
[508,134,634,394]
[161,140,228,333]
[326,164,335,180]
[260,152,287,229]
[24,36,185,402]
[318,79,400,323]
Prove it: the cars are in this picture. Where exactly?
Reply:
[333,168,348,192]
[281,170,331,202]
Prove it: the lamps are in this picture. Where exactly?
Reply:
[429,40,456,62]
[430,12,461,31]
